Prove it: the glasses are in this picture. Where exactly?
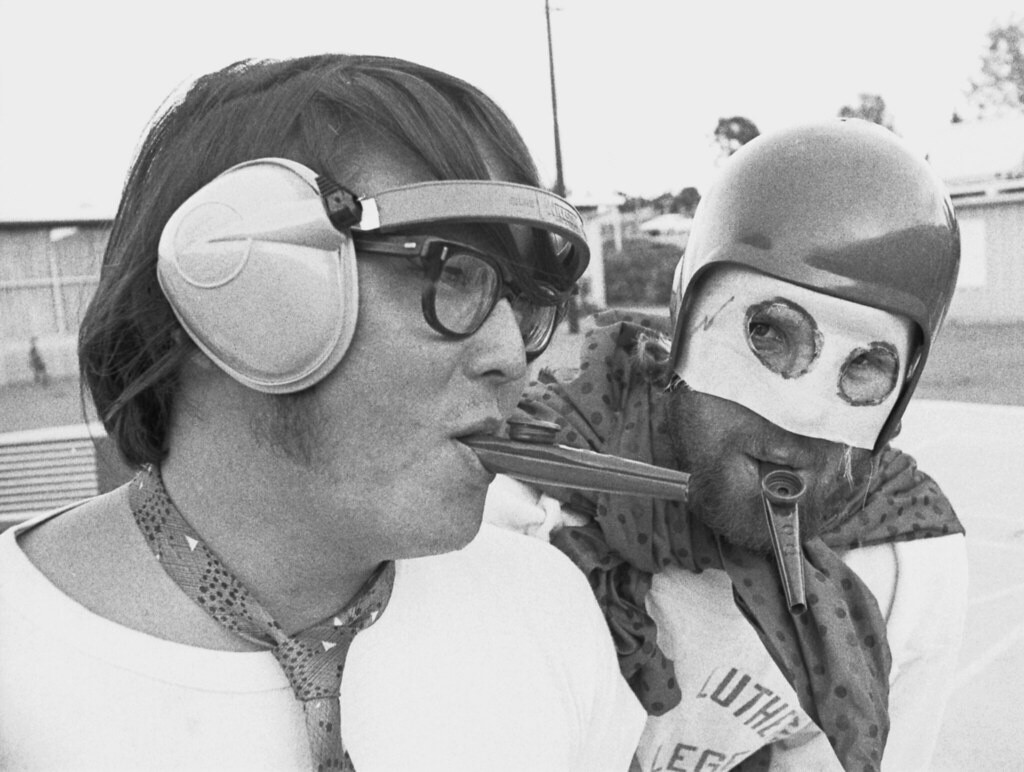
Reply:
[350,229,570,364]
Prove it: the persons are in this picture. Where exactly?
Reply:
[0,54,649,772]
[506,114,967,772]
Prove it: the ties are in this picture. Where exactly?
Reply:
[127,458,396,772]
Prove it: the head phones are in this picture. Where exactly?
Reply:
[157,155,591,395]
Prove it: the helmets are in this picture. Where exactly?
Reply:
[662,117,961,457]
